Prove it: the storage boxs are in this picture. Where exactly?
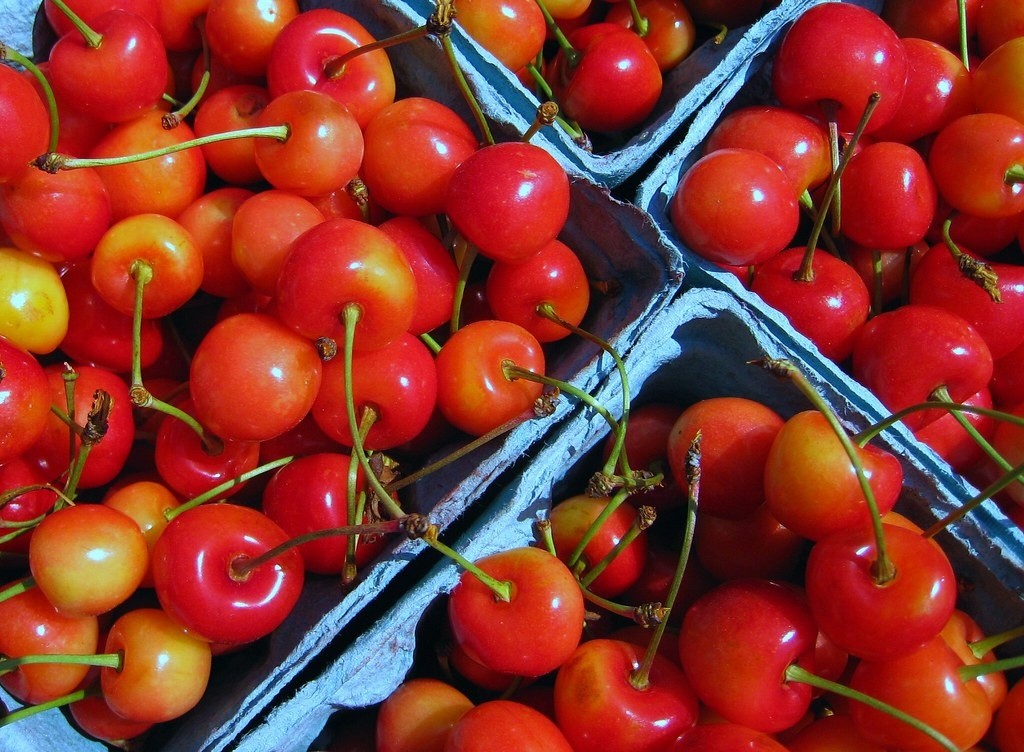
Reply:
[0,0,1022,752]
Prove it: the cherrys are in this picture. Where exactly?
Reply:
[0,1,1024,752]
